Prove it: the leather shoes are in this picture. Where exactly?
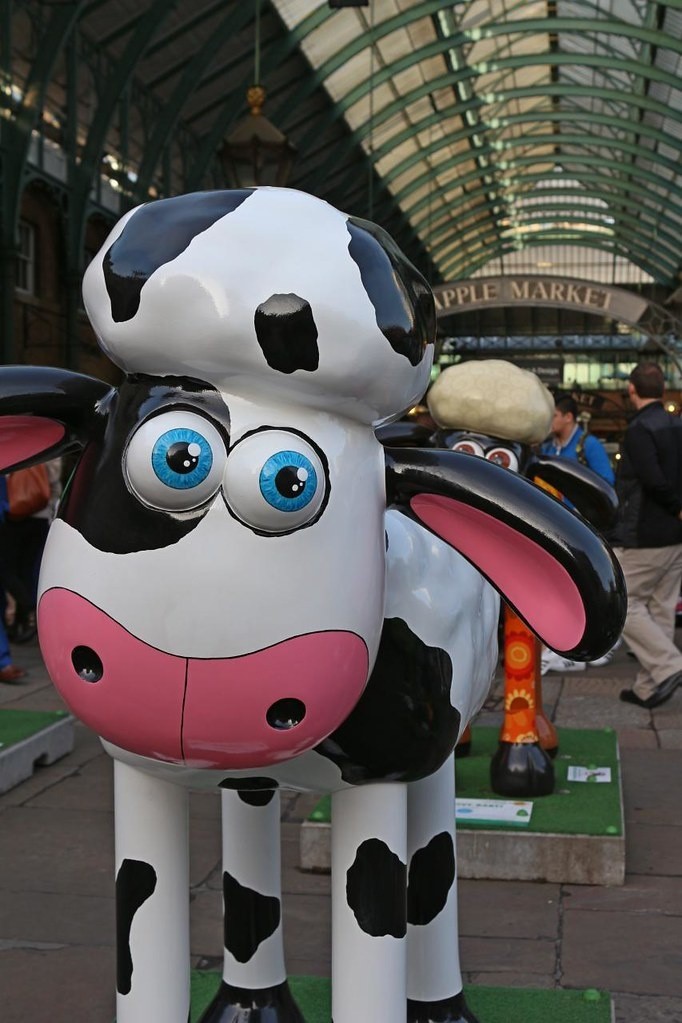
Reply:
[1,663,28,682]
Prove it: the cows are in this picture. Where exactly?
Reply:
[2,183,630,1022]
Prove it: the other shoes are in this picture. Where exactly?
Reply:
[541,639,619,676]
[620,689,645,707]
[644,669,682,709]
[626,649,638,662]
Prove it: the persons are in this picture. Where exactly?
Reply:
[608,362,682,713]
[535,396,622,676]
[0,575,30,685]
